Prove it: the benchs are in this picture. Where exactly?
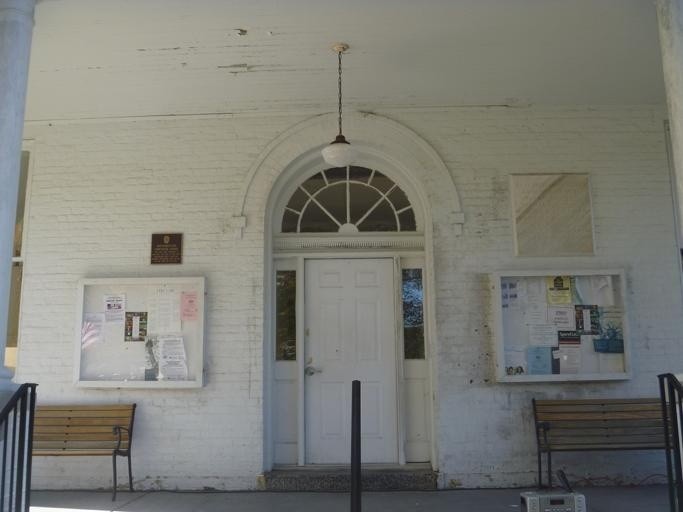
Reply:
[531,396,673,491]
[32,403,136,502]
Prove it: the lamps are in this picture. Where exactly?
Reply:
[320,48,359,167]
